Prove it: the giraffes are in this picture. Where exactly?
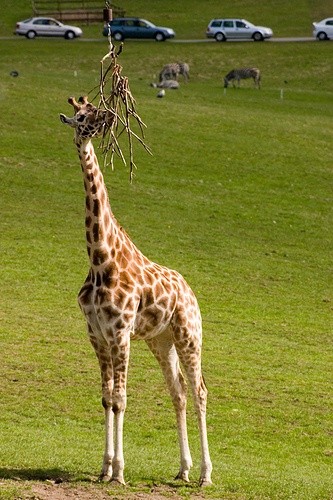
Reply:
[59,96,213,488]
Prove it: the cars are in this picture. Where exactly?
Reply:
[16,18,82,39]
[313,18,333,40]
[206,19,272,42]
[103,19,174,41]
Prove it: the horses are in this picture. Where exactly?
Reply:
[223,66,261,90]
[149,79,179,90]
[157,62,179,83]
[177,62,190,80]
